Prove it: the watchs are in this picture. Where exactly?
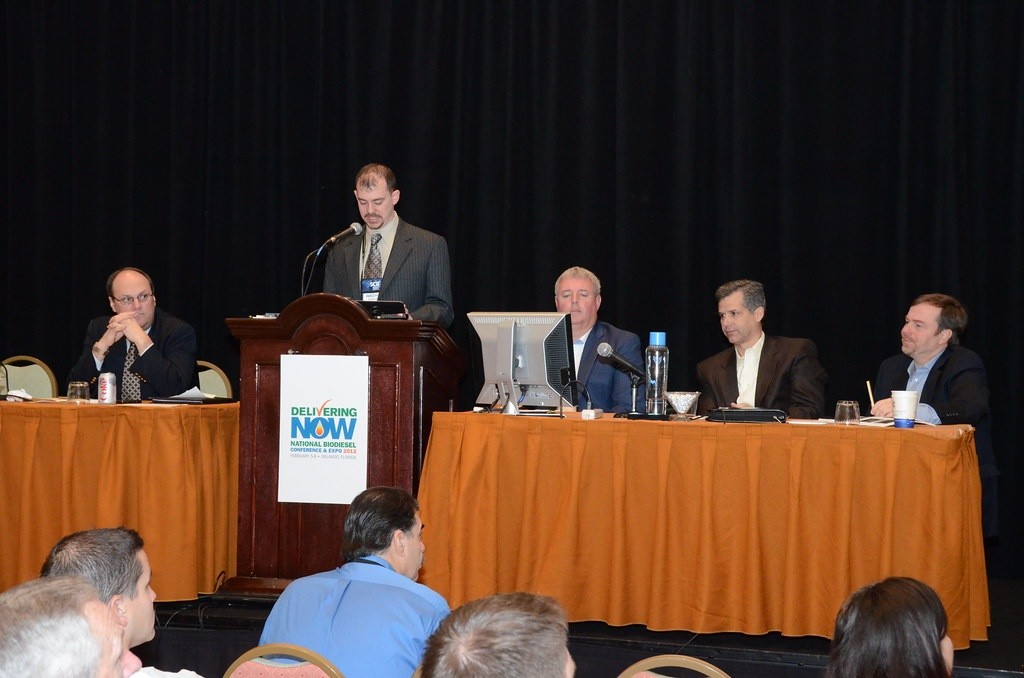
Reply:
[93,340,109,356]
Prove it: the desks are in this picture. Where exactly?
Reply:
[0,398,242,603]
[417,409,992,651]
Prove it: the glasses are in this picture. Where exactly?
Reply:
[112,293,153,305]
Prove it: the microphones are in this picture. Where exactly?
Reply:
[326,222,362,245]
[597,342,646,378]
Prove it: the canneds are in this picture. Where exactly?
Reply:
[98,372,117,404]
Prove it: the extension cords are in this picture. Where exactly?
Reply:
[581,408,603,420]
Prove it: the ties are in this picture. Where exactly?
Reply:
[120,343,141,403]
[364,233,382,278]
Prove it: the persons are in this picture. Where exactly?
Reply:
[866,293,1001,539]
[553,267,647,413]
[694,279,828,419]
[63,267,200,401]
[825,577,955,678]
[257,487,452,678]
[419,591,577,678]
[0,525,205,678]
[322,163,454,327]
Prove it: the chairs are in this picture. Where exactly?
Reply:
[0,356,59,399]
[617,654,731,678]
[197,360,232,398]
[222,643,346,678]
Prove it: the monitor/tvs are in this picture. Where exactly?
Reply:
[356,300,406,319]
[466,312,578,416]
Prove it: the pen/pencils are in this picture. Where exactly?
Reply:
[866,381,874,406]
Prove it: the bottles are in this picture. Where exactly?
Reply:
[644,332,669,416]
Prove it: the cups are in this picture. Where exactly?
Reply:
[66,382,91,405]
[663,391,702,417]
[833,400,860,424]
[890,390,919,429]
